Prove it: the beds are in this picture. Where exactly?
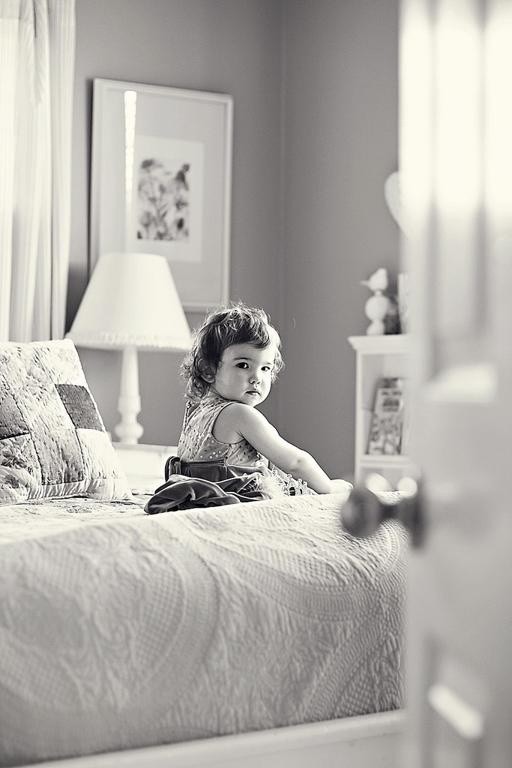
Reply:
[0,487,410,768]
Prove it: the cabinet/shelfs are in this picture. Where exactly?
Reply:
[348,335,410,489]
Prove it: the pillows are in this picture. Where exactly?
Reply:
[1,337,134,504]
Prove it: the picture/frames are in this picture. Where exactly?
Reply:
[90,78,232,314]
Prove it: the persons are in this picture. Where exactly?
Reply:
[162,296,354,502]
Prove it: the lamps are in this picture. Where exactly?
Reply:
[64,252,197,444]
